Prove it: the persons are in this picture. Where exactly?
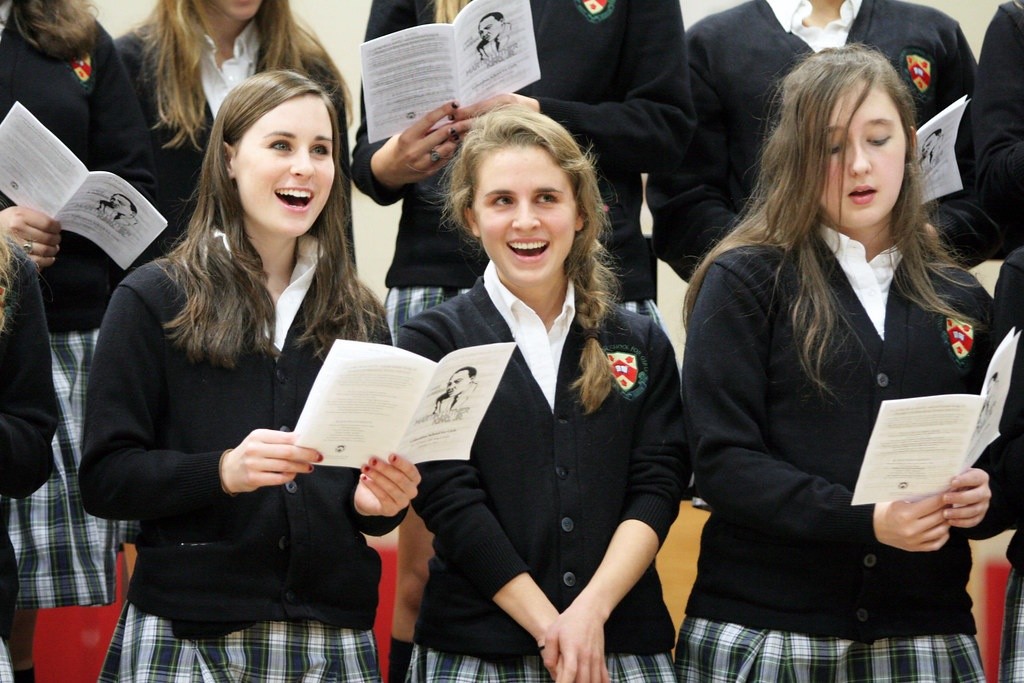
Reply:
[396,103,687,683]
[645,0,1024,282]
[682,42,1024,683]
[0,0,161,683]
[0,219,59,683]
[112,0,358,581]
[352,0,698,683]
[80,70,421,683]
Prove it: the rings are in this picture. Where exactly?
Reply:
[430,150,440,162]
